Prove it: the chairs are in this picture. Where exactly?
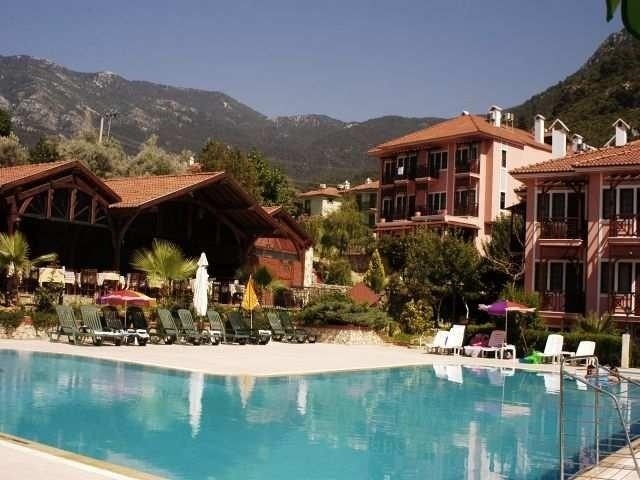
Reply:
[47,305,320,346]
[423,324,596,368]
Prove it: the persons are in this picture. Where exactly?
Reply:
[584,365,598,378]
[606,367,622,384]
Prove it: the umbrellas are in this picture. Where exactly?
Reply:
[241,276,261,329]
[185,372,206,437]
[481,300,536,343]
[193,251,209,328]
[95,361,148,398]
[474,373,530,418]
[238,376,254,406]
[98,289,157,329]
